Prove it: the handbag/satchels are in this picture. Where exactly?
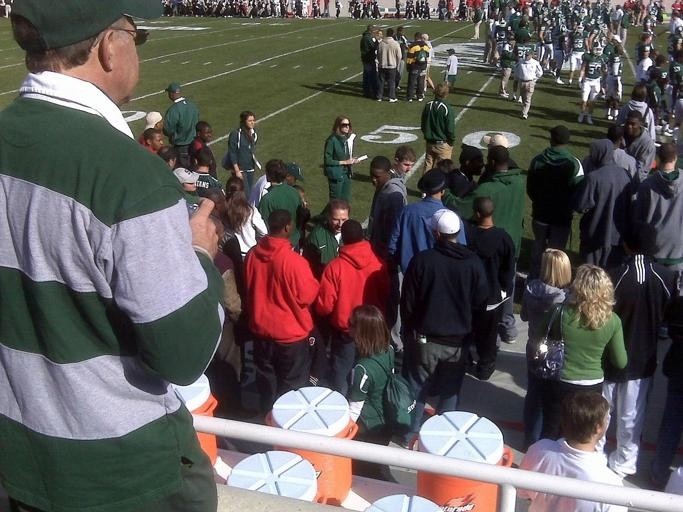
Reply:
[532,304,565,380]
[222,152,240,169]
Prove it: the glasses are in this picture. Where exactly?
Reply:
[342,124,349,127]
[124,29,146,45]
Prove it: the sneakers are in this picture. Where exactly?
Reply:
[522,114,529,119]
[606,115,619,120]
[377,94,425,103]
[656,124,673,136]
[477,337,515,380]
[499,92,522,102]
[556,78,572,85]
[578,113,592,125]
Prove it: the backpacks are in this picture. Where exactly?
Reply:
[372,350,416,426]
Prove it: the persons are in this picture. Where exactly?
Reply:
[0,0,16,21]
[137,0,682,512]
[0,1,225,511]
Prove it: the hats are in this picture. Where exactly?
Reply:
[165,83,181,94]
[173,168,199,184]
[490,134,508,148]
[426,209,460,234]
[418,169,447,195]
[11,0,163,52]
[286,164,304,181]
[145,112,162,130]
